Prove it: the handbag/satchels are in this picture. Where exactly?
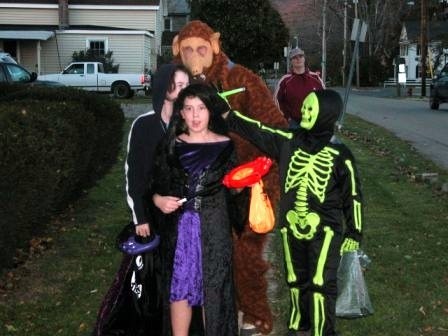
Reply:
[335,249,376,318]
[249,179,275,234]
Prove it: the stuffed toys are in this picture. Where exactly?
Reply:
[171,20,291,336]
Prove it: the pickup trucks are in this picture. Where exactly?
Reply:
[38,62,152,100]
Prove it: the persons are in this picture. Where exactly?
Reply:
[217,88,362,336]
[273,48,325,133]
[96,63,252,336]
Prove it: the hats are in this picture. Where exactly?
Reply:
[289,48,305,60]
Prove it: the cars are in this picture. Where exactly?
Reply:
[0,60,66,88]
[430,62,448,110]
